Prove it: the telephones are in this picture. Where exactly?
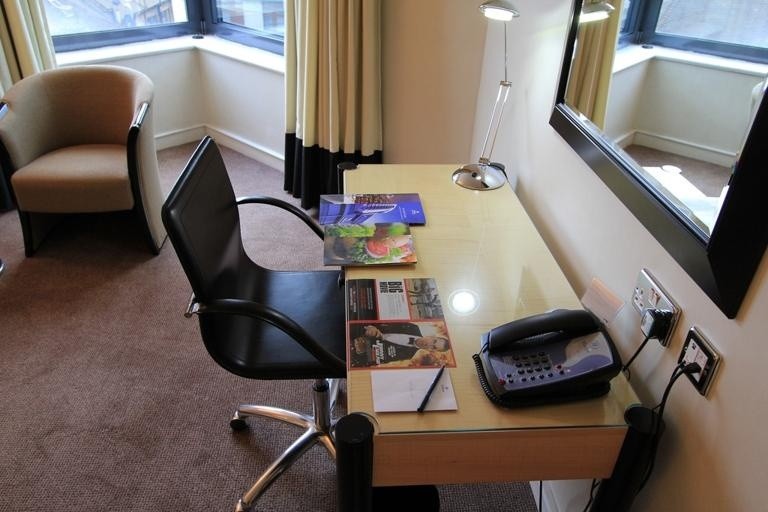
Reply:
[479,309,622,407]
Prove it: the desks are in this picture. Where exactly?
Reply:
[331,158,669,511]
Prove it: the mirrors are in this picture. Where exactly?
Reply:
[541,1,768,324]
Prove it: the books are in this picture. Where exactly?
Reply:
[370,370,458,413]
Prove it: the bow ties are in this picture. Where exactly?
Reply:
[407,337,416,345]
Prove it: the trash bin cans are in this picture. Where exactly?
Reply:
[375,485,440,512]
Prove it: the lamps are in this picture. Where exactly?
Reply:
[450,1,518,193]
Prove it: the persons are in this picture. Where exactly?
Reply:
[349,322,450,368]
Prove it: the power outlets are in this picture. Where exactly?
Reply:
[627,266,680,348]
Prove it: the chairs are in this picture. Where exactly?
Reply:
[161,132,350,511]
[0,65,176,258]
